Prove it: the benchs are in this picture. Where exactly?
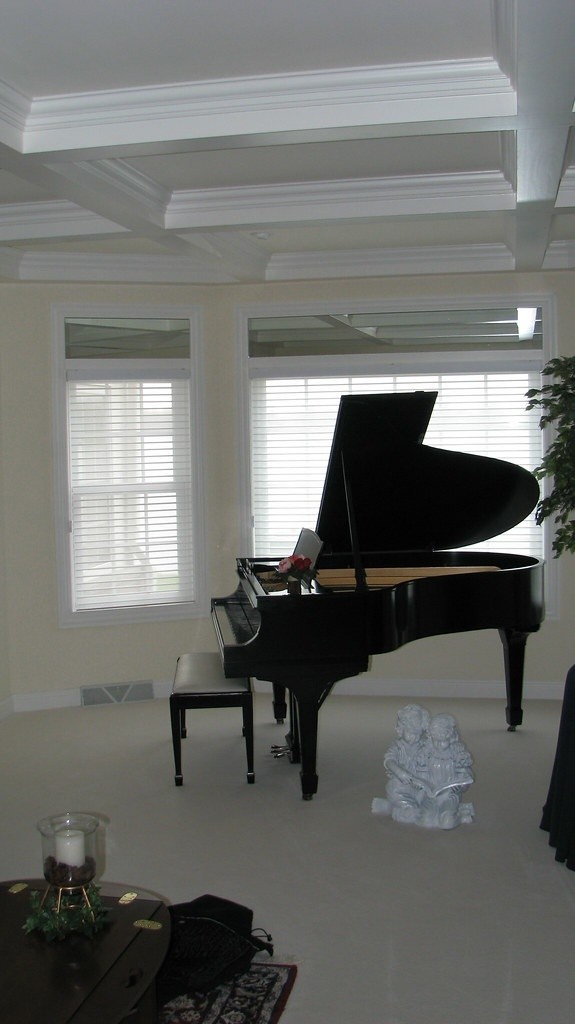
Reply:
[169,652,255,786]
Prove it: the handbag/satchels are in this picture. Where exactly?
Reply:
[157,893,274,1006]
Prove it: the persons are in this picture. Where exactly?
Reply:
[371,703,475,830]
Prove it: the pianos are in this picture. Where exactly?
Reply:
[210,391,546,800]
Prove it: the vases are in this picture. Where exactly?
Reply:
[288,575,301,596]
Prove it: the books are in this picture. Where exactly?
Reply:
[408,778,473,799]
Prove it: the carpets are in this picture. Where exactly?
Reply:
[156,963,298,1024]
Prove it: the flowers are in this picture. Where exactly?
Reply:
[272,555,319,593]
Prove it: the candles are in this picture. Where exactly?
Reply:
[56,830,86,868]
